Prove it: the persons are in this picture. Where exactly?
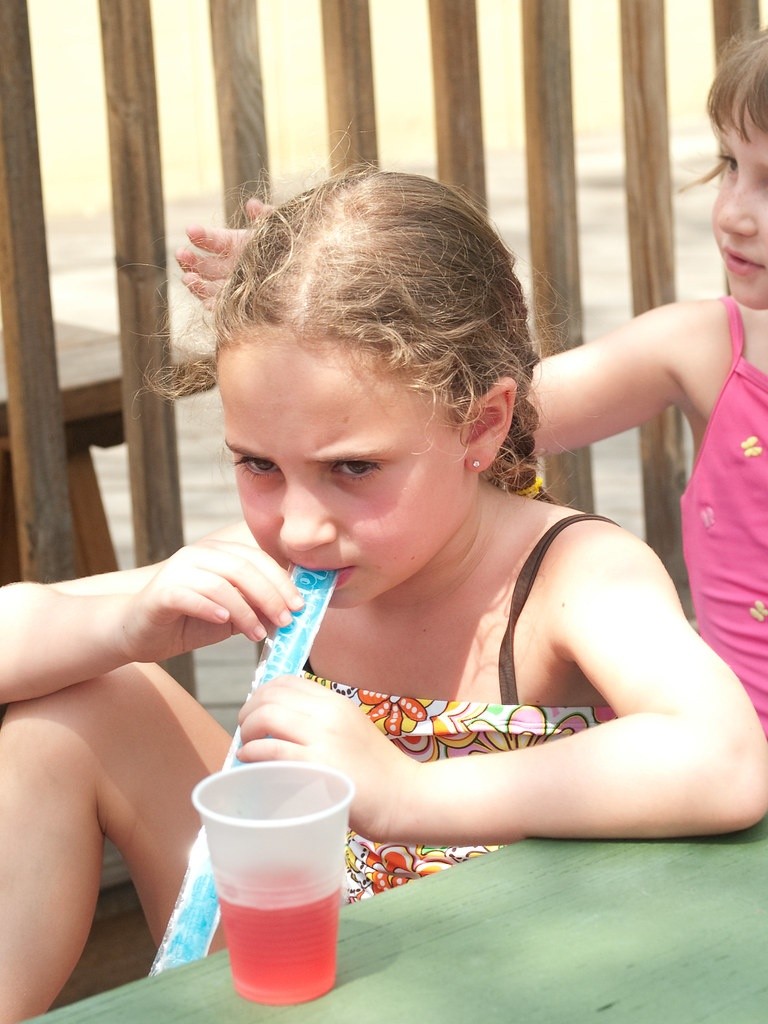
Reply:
[175,23,768,740]
[0,167,768,1024]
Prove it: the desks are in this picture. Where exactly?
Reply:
[2,314,217,581]
[22,809,767,1023]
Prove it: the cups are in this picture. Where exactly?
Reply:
[192,762,355,1004]
